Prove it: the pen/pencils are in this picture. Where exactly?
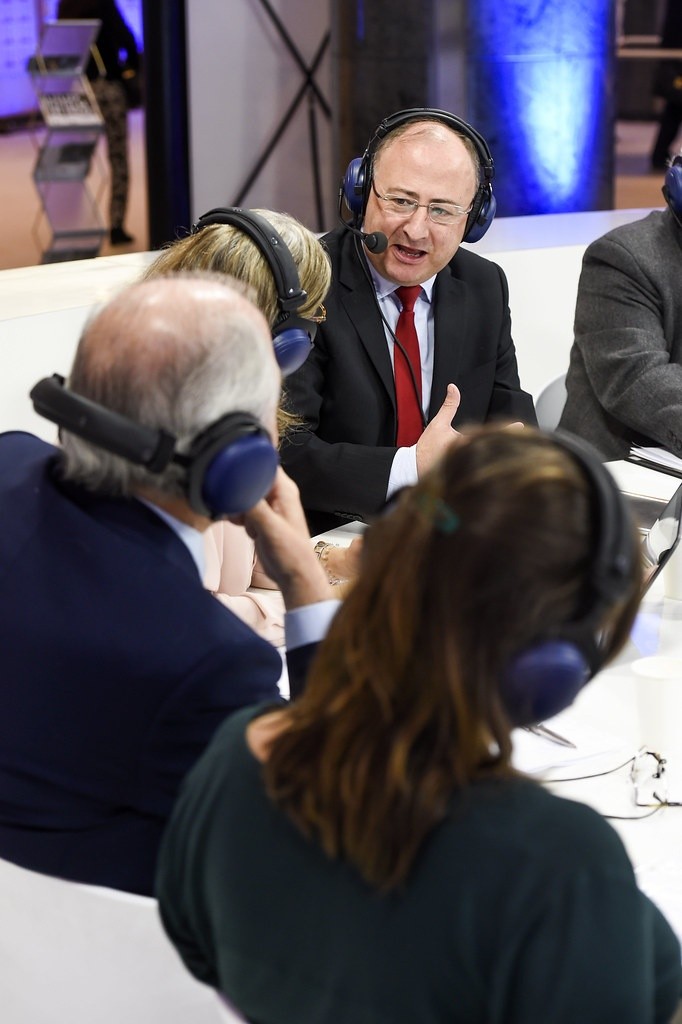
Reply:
[531,724,575,749]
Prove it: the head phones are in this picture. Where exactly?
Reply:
[337,106,496,243]
[662,149,682,227]
[362,427,628,730]
[29,374,278,519]
[192,206,317,379]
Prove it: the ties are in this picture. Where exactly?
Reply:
[394,284,425,449]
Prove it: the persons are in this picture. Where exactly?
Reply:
[1,277,338,899]
[136,205,333,647]
[153,430,682,1024]
[277,120,542,527]
[55,0,141,247]
[554,155,682,464]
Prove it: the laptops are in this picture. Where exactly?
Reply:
[640,483,682,599]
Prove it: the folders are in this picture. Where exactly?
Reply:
[624,445,682,479]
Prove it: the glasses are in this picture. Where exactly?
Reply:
[665,154,682,169]
[308,303,327,325]
[371,175,474,225]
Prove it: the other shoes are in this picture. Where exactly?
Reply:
[110,226,135,247]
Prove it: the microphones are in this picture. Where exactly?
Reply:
[337,177,388,254]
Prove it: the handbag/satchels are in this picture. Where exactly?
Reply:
[120,52,148,110]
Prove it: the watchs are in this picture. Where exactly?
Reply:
[313,542,338,588]
